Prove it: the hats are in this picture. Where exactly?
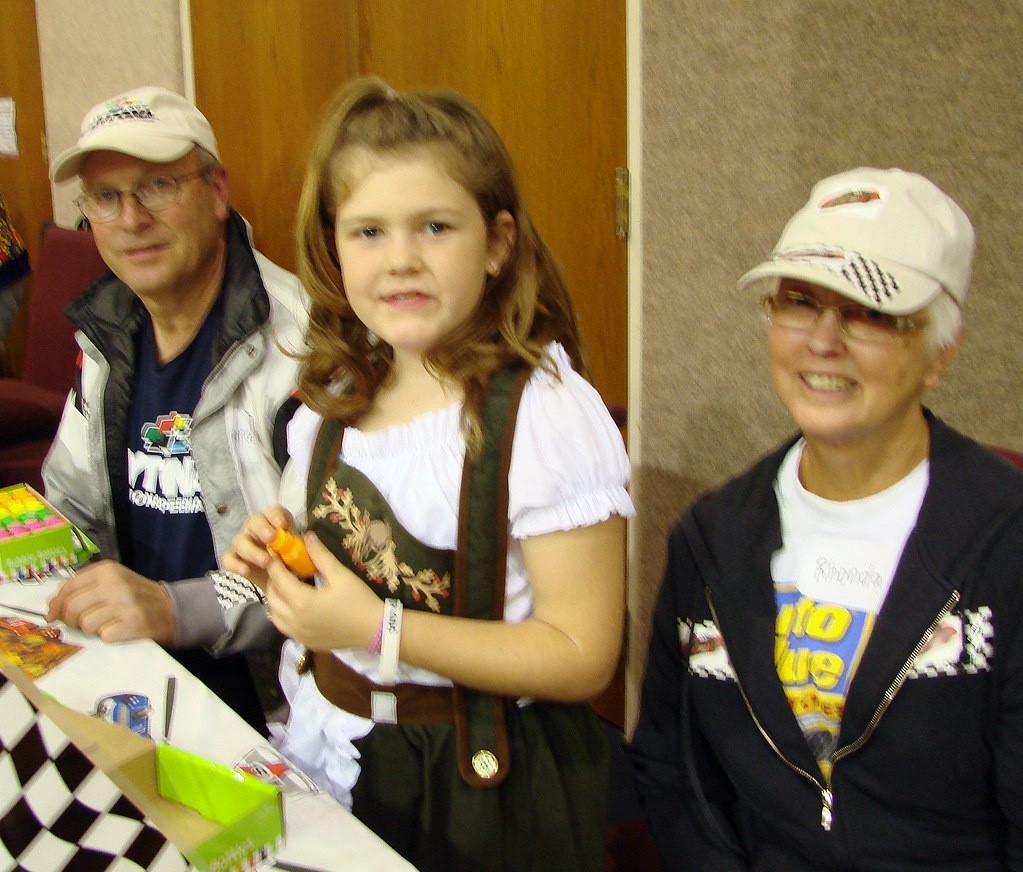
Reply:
[736,166,977,315]
[48,86,220,185]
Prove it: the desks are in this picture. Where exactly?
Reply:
[0,482,419,872]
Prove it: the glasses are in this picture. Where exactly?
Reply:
[759,288,951,345]
[72,163,213,224]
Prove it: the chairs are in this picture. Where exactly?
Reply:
[0,223,109,487]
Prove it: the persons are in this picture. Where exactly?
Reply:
[41,86,316,738]
[630,167,1023,872]
[220,79,637,872]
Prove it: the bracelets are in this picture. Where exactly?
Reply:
[370,599,403,687]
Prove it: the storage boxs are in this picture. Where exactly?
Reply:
[7,664,287,872]
